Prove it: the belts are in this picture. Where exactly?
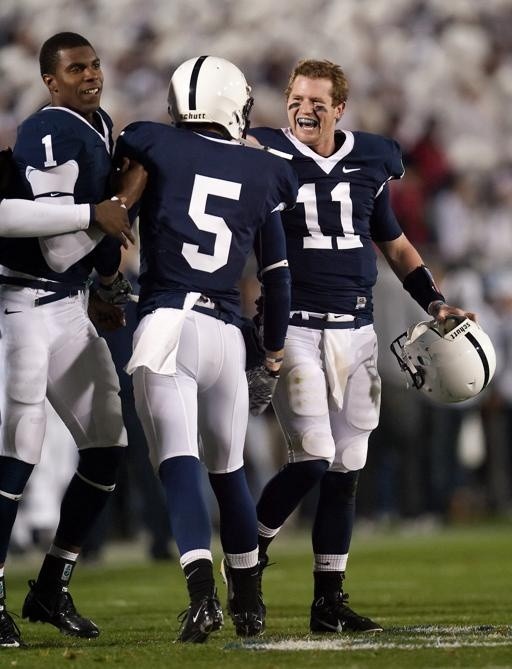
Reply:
[289,315,370,329]
[191,305,241,328]
[1,275,80,308]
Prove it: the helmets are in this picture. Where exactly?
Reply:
[390,316,497,404]
[167,57,253,144]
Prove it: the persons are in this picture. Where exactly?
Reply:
[1,30,151,653]
[86,52,300,648]
[0,193,136,251]
[219,57,482,636]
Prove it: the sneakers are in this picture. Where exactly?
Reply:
[22,580,100,638]
[310,602,382,635]
[220,557,269,636]
[0,577,24,647]
[176,599,221,643]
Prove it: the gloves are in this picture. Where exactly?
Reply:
[247,365,279,415]
[90,272,132,326]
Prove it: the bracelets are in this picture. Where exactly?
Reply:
[402,265,446,315]
